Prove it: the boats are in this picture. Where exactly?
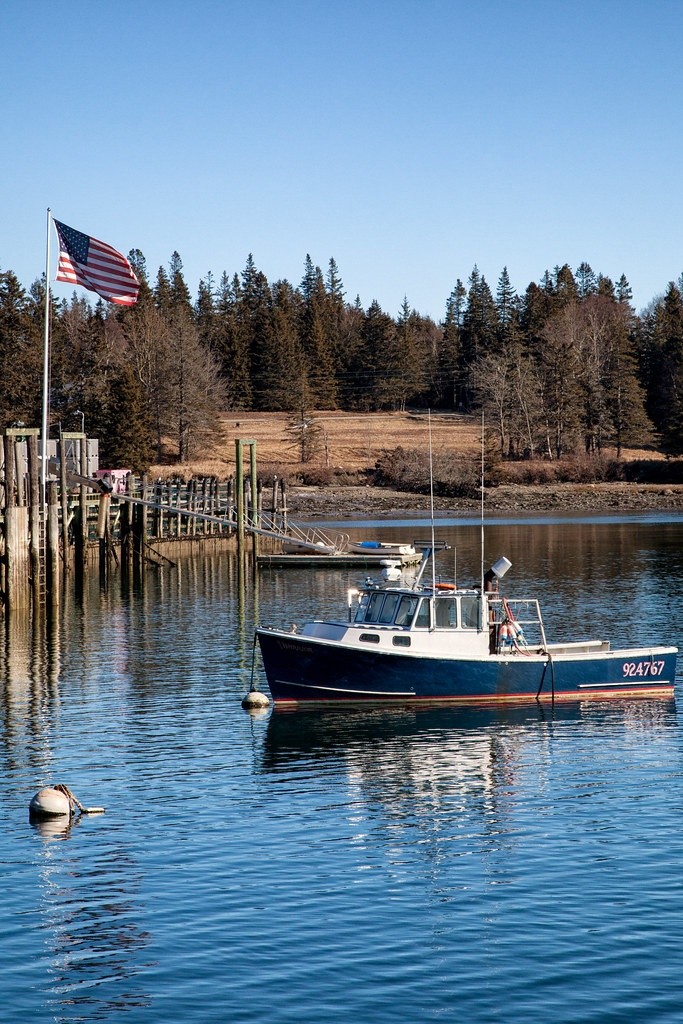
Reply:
[282,543,335,555]
[347,541,415,555]
[254,408,678,712]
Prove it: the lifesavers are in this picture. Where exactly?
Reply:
[430,584,456,590]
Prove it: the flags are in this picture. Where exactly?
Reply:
[53,218,141,307]
